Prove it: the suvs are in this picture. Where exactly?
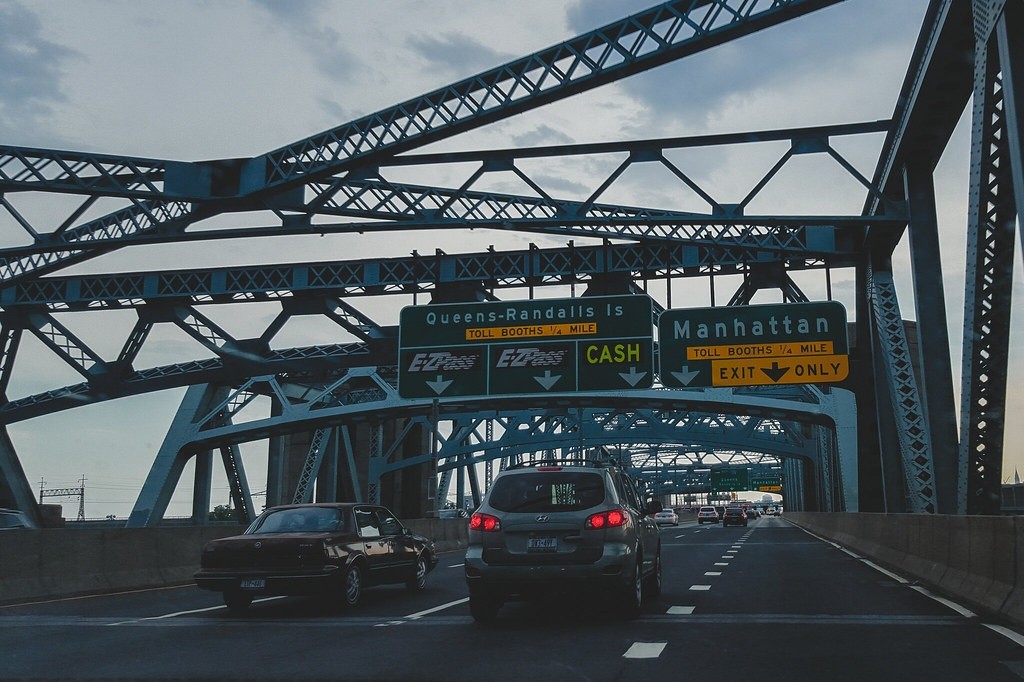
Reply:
[698,505,719,524]
[465,458,662,619]
[723,508,747,527]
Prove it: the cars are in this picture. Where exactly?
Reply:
[715,506,726,519]
[441,499,466,518]
[655,508,679,526]
[193,503,439,610]
[741,507,783,519]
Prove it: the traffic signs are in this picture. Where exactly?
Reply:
[395,295,654,400]
[657,300,850,390]
